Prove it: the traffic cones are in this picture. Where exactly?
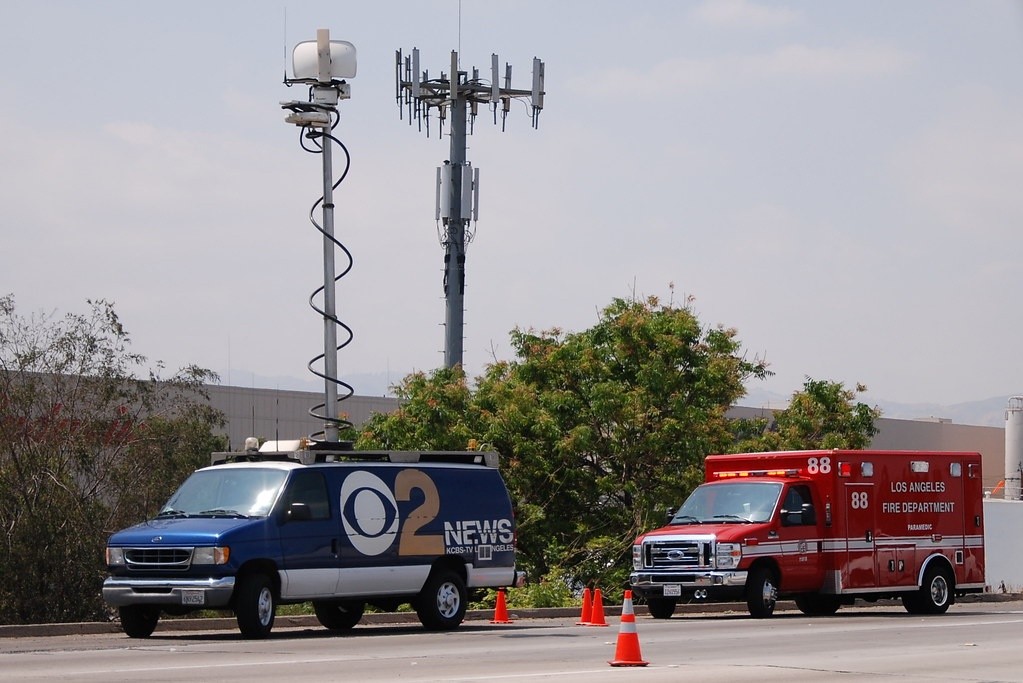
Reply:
[575,587,594,626]
[489,587,515,624]
[607,590,650,668]
[584,589,611,626]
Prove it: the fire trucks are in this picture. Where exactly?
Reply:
[628,448,992,621]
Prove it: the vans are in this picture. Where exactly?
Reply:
[101,438,516,640]
